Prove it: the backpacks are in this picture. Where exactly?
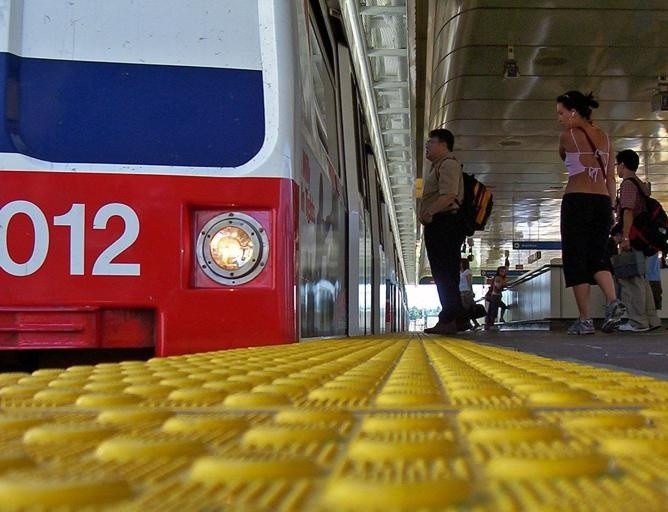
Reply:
[438,158,495,238]
[620,177,668,260]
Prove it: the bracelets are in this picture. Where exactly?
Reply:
[622,237,629,240]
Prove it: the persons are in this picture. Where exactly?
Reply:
[483,266,502,330]
[459,259,476,329]
[486,265,508,329]
[555,88,627,336]
[416,129,465,335]
[467,316,480,330]
[610,149,666,333]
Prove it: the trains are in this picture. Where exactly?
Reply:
[1,0,409,358]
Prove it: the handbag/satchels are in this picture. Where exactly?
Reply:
[466,301,488,319]
[484,285,492,301]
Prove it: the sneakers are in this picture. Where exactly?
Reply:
[618,315,663,331]
[566,317,595,334]
[600,300,629,332]
[424,319,470,335]
